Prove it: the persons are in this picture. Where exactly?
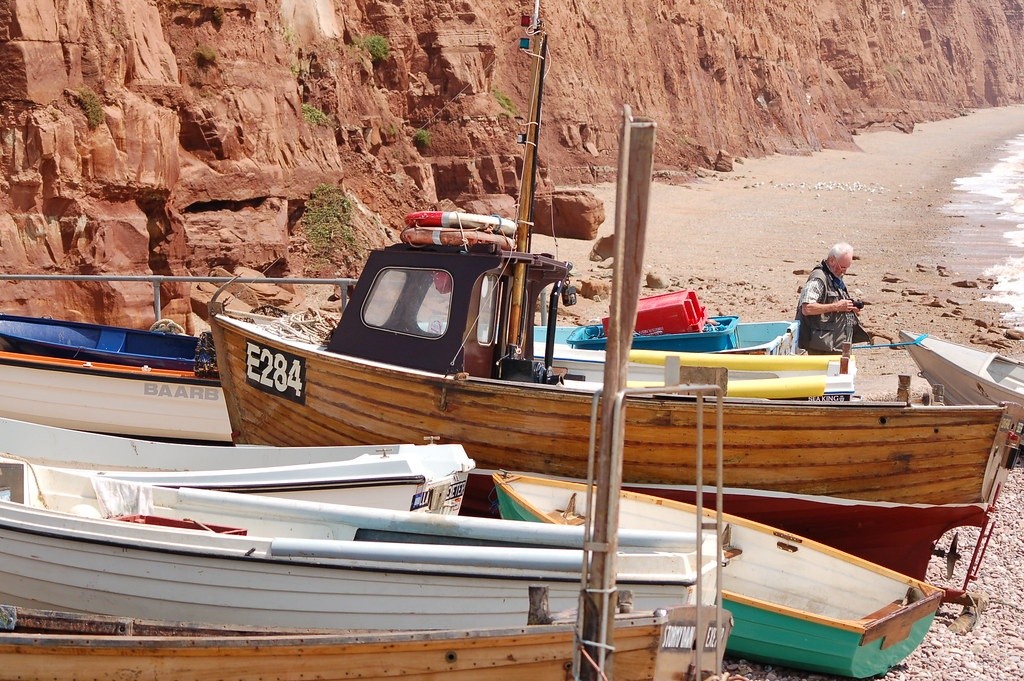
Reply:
[795,242,871,355]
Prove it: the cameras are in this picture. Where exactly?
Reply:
[848,298,864,309]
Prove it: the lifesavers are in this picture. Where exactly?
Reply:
[400,226,517,251]
[404,210,518,236]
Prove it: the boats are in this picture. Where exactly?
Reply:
[0,0,1011,681]
[488,470,948,679]
[897,328,1024,451]
